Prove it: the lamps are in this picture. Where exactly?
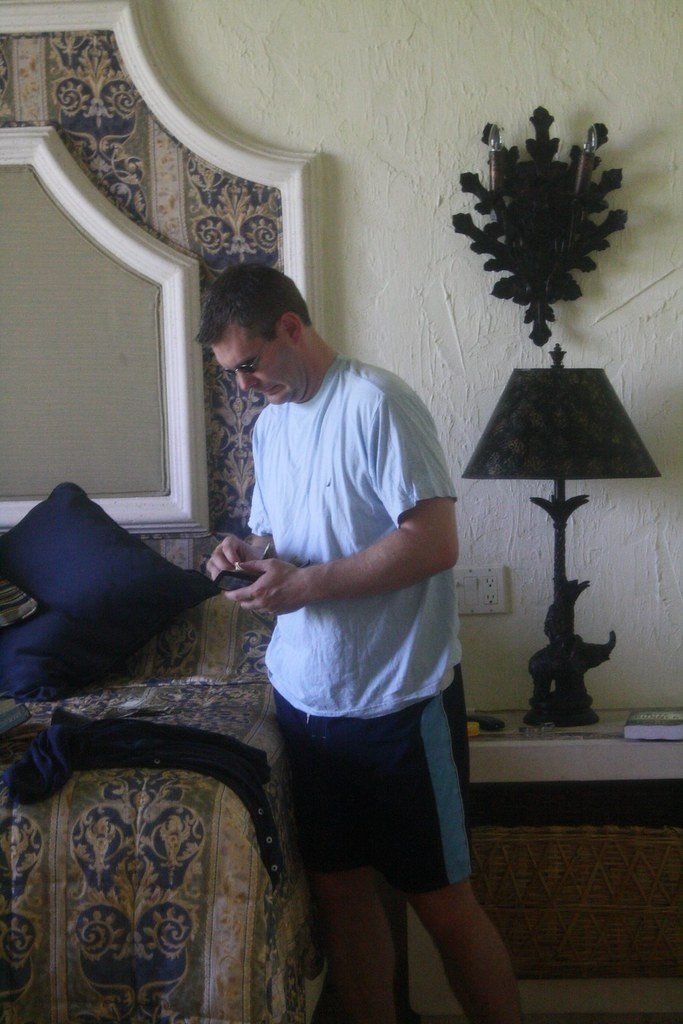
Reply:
[462,344,662,728]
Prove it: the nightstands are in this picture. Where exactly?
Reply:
[406,728,683,1024]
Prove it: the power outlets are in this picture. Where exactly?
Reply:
[452,564,506,615]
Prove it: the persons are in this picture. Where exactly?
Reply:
[195,262,523,1024]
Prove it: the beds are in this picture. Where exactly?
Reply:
[0,0,324,1024]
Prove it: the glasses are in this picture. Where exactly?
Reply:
[224,319,274,376]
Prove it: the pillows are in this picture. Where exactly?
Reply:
[0,482,220,699]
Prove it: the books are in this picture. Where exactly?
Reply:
[623,706,683,740]
